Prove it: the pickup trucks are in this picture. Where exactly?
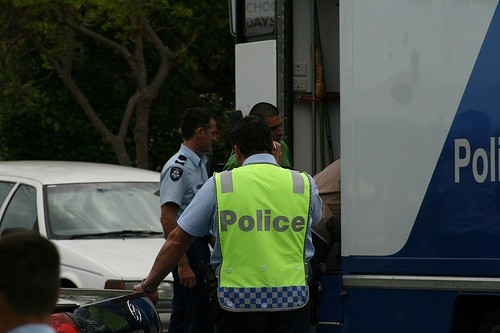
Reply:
[50,287,162,333]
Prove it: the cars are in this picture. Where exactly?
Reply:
[0,160,176,333]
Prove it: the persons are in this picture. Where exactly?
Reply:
[0,226,61,333]
[132,101,324,333]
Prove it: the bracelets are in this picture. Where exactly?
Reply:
[140,278,157,291]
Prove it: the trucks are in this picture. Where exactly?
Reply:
[234,0,500,333]
